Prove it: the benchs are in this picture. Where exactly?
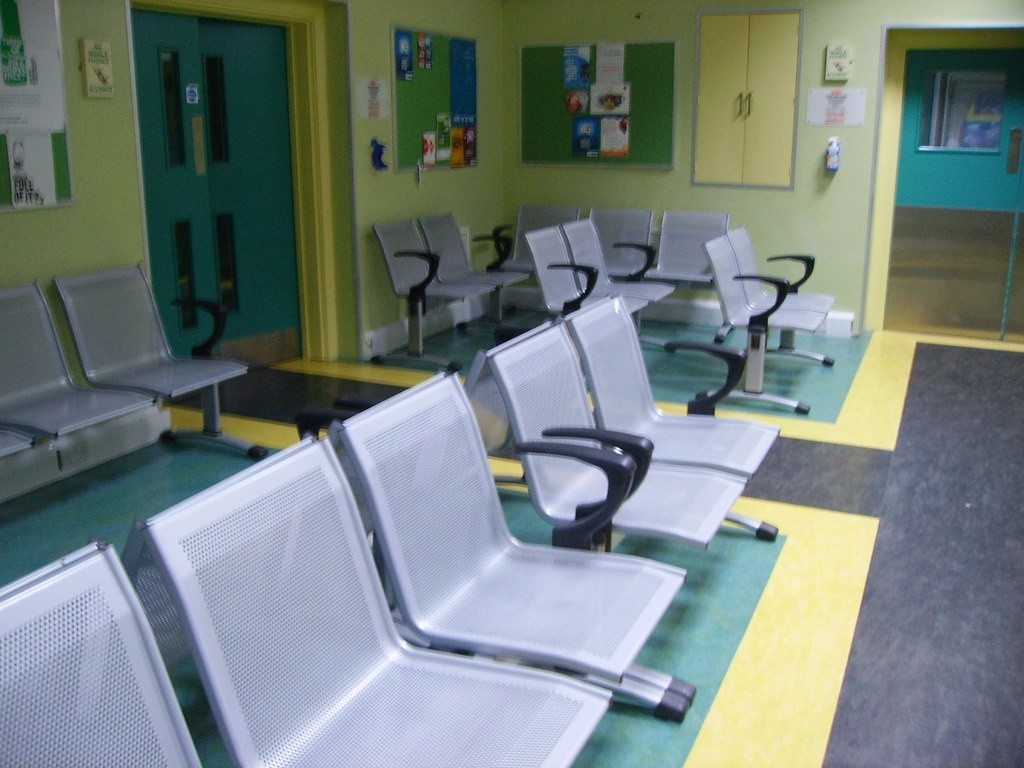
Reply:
[0,205,836,767]
